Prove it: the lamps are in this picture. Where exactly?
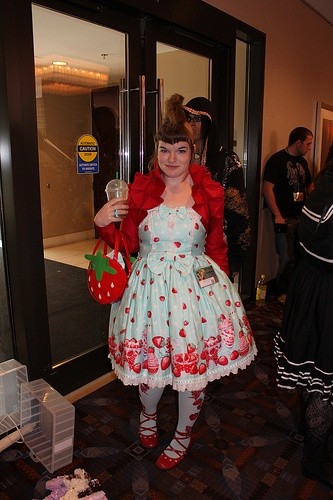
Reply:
[35,65,109,97]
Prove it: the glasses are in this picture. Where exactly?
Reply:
[186,116,202,123]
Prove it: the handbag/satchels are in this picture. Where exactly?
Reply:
[84,224,132,305]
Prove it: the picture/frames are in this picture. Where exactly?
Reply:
[313,102,333,178]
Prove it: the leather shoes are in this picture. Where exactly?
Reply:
[155,430,193,472]
[137,409,159,449]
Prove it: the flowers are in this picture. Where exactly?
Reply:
[43,468,108,500]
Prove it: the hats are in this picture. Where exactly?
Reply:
[182,96,216,122]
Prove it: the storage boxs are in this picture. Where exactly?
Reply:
[20,378,75,473]
[0,359,31,435]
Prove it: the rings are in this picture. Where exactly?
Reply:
[114,210,118,217]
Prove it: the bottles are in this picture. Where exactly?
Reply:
[255,275,266,306]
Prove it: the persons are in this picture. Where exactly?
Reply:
[261,126,315,304]
[93,93,258,470]
[273,143,333,488]
[147,97,251,284]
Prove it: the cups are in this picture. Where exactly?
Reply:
[105,180,130,220]
